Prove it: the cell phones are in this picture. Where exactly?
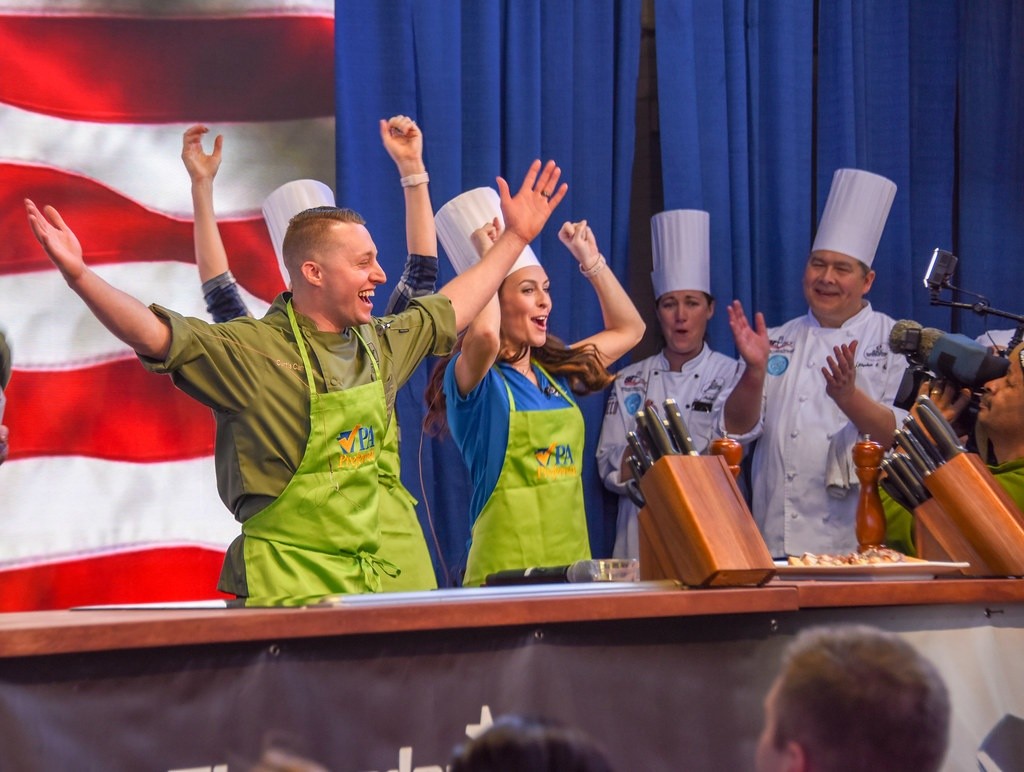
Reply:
[692,401,712,412]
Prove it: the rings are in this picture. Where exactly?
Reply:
[541,191,553,200]
[932,390,942,396]
[412,120,416,124]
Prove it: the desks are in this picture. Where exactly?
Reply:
[0,562,1024,772]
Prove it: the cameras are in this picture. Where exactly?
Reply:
[889,319,1011,467]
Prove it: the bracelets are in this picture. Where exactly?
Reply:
[579,253,607,279]
[400,172,431,189]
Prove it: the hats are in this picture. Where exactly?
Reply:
[260,178,333,292]
[810,169,897,269]
[648,210,711,300]
[435,186,543,282]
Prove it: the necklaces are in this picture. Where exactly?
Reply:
[522,365,532,378]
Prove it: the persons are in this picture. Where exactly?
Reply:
[595,209,751,564]
[753,619,953,772]
[420,187,648,594]
[709,169,937,564]
[450,721,620,772]
[877,341,1024,560]
[22,158,570,610]
[182,112,439,320]
